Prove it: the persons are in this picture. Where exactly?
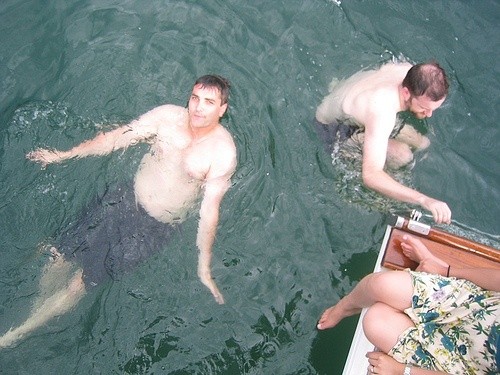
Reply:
[1,74,238,352]
[314,234,500,375]
[313,58,453,227]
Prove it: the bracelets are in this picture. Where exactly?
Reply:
[447,264,451,278]
[403,363,412,375]
